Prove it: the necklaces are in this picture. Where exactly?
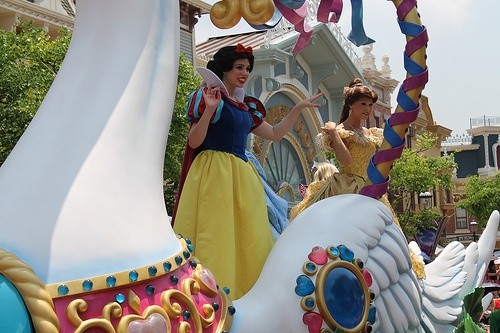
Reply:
[347,122,363,137]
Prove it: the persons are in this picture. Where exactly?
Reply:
[313,163,337,182]
[417,231,436,263]
[289,78,425,280]
[172,43,325,303]
[484,259,500,311]
